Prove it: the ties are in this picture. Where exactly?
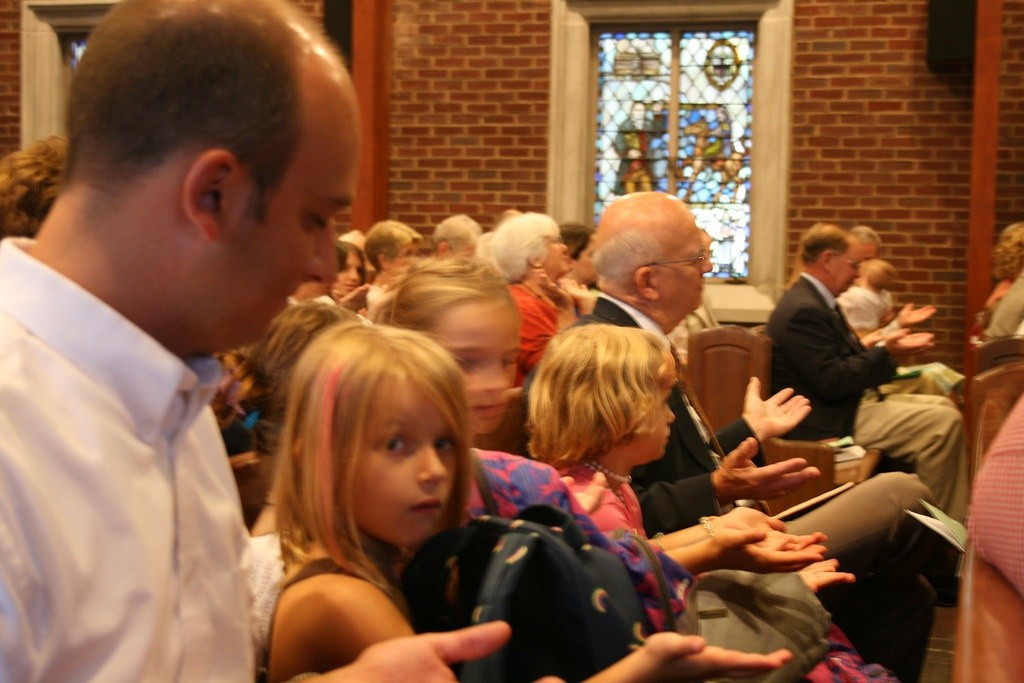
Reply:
[669,340,772,517]
[834,303,867,353]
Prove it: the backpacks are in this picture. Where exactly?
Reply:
[398,503,656,683]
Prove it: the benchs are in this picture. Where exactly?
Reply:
[689,325,880,513]
[221,388,528,533]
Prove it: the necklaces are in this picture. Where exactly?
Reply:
[584,460,632,508]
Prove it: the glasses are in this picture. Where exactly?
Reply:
[646,247,713,266]
[832,251,863,269]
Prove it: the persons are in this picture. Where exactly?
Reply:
[1,191,1023,683]
[0,0,362,683]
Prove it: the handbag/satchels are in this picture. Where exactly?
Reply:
[670,569,831,683]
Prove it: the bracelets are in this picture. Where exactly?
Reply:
[698,516,715,537]
